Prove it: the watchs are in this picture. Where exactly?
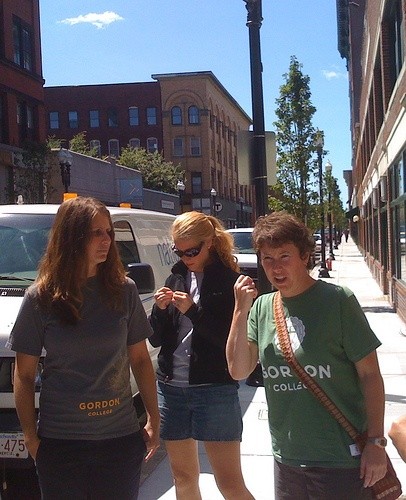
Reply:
[366,436,387,448]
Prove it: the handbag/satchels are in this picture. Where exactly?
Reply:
[355,429,402,500]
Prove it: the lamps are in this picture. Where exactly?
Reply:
[372,175,388,209]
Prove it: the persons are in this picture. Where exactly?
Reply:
[147,211,256,500]
[226,212,387,500]
[343,229,349,242]
[4,197,161,500]
[388,414,406,463]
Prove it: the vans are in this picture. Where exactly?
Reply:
[0,203,201,474]
[224,228,258,279]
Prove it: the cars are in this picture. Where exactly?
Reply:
[312,226,338,252]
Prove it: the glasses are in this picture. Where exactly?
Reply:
[171,241,205,258]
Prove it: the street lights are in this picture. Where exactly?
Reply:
[239,195,245,227]
[176,180,185,214]
[210,187,217,216]
[324,158,336,260]
[313,126,330,279]
